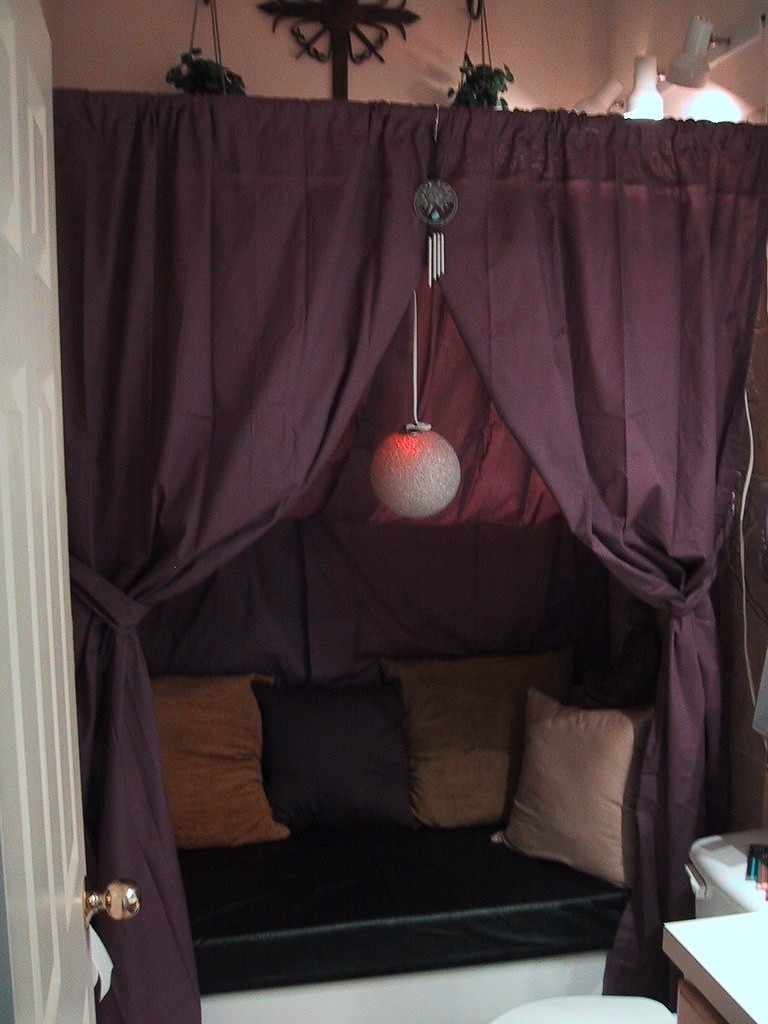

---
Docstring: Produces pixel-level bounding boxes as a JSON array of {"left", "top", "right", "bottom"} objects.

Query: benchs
[{"left": 178, "top": 849, "right": 632, "bottom": 1024}]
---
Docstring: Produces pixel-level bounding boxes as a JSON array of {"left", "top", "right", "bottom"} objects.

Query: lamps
[
  {"left": 371, "top": 290, "right": 461, "bottom": 515},
  {"left": 665, "top": 15, "right": 730, "bottom": 92},
  {"left": 628, "top": 55, "right": 668, "bottom": 122}
]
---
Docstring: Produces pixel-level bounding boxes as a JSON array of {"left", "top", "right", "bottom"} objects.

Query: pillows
[
  {"left": 253, "top": 672, "right": 415, "bottom": 830},
  {"left": 492, "top": 686, "right": 655, "bottom": 889},
  {"left": 150, "top": 673, "right": 290, "bottom": 848},
  {"left": 378, "top": 652, "right": 567, "bottom": 830}
]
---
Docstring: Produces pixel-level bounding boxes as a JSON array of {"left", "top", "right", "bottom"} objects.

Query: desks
[{"left": 662, "top": 912, "right": 768, "bottom": 1024}]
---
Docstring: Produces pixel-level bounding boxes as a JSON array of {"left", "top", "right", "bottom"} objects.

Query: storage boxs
[{"left": 684, "top": 828, "right": 768, "bottom": 920}]
